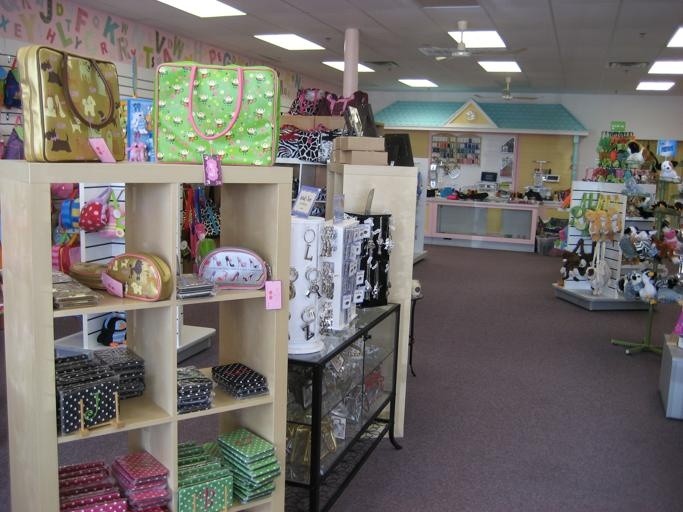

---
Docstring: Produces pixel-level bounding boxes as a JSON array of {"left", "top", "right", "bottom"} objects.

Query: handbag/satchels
[
  {"left": 289, "top": 86, "right": 324, "bottom": 115},
  {"left": 559, "top": 239, "right": 595, "bottom": 281},
  {"left": 198, "top": 246, "right": 272, "bottom": 292},
  {"left": 107, "top": 250, "right": 174, "bottom": 304},
  {"left": 193, "top": 188, "right": 227, "bottom": 240},
  {"left": 153, "top": 60, "right": 282, "bottom": 167},
  {"left": 290, "top": 416, "right": 337, "bottom": 465},
  {"left": 342, "top": 90, "right": 378, "bottom": 139},
  {"left": 18, "top": 42, "right": 130, "bottom": 161},
  {"left": 586, "top": 242, "right": 612, "bottom": 295}
]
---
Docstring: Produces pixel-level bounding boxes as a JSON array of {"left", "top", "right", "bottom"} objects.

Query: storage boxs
[
  {"left": 658, "top": 333, "right": 683, "bottom": 421},
  {"left": 280, "top": 115, "right": 315, "bottom": 131},
  {"left": 315, "top": 116, "right": 348, "bottom": 130}
]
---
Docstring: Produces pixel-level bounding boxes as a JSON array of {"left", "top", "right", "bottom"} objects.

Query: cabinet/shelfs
[
  {"left": 325, "top": 162, "right": 418, "bottom": 439},
  {"left": 285, "top": 302, "right": 402, "bottom": 512},
  {"left": 0, "top": 160, "right": 293, "bottom": 512},
  {"left": 423, "top": 197, "right": 539, "bottom": 252},
  {"left": 552, "top": 180, "right": 659, "bottom": 312}
]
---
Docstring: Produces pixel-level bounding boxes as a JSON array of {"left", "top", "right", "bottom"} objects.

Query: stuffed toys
[
  {"left": 560, "top": 238, "right": 610, "bottom": 295},
  {"left": 626, "top": 273, "right": 643, "bottom": 300},
  {"left": 591, "top": 167, "right": 624, "bottom": 184},
  {"left": 663, "top": 226, "right": 680, "bottom": 260},
  {"left": 620, "top": 227, "right": 638, "bottom": 263},
  {"left": 659, "top": 193, "right": 683, "bottom": 216},
  {"left": 639, "top": 197, "right": 655, "bottom": 219},
  {"left": 634, "top": 230, "right": 661, "bottom": 263},
  {"left": 658, "top": 279, "right": 683, "bottom": 306},
  {"left": 639, "top": 270, "right": 657, "bottom": 306},
  {"left": 661, "top": 162, "right": 680, "bottom": 179},
  {"left": 617, "top": 275, "right": 629, "bottom": 291},
  {"left": 627, "top": 194, "right": 641, "bottom": 218},
  {"left": 570, "top": 194, "right": 621, "bottom": 244},
  {"left": 624, "top": 180, "right": 640, "bottom": 195}
]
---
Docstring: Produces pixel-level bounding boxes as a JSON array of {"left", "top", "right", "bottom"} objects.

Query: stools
[{"left": 408, "top": 280, "right": 424, "bottom": 377}]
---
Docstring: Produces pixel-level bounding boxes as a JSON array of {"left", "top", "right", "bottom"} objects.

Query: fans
[
  {"left": 481, "top": 76, "right": 544, "bottom": 101},
  {"left": 417, "top": 19, "right": 527, "bottom": 64}
]
